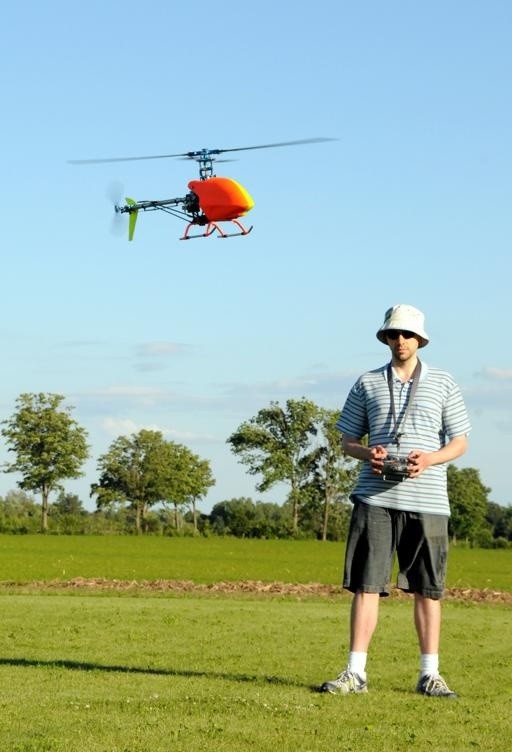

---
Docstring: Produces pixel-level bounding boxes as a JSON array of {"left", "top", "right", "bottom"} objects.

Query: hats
[{"left": 376, "top": 304, "right": 429, "bottom": 348}]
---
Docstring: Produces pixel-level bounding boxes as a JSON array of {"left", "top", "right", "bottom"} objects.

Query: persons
[{"left": 317, "top": 302, "right": 474, "bottom": 697}]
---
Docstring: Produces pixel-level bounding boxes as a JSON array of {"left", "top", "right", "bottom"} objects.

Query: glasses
[{"left": 386, "top": 330, "right": 414, "bottom": 339}]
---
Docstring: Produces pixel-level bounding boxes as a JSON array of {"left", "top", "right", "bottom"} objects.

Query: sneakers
[
  {"left": 320, "top": 670, "right": 367, "bottom": 695},
  {"left": 415, "top": 675, "right": 455, "bottom": 696}
]
